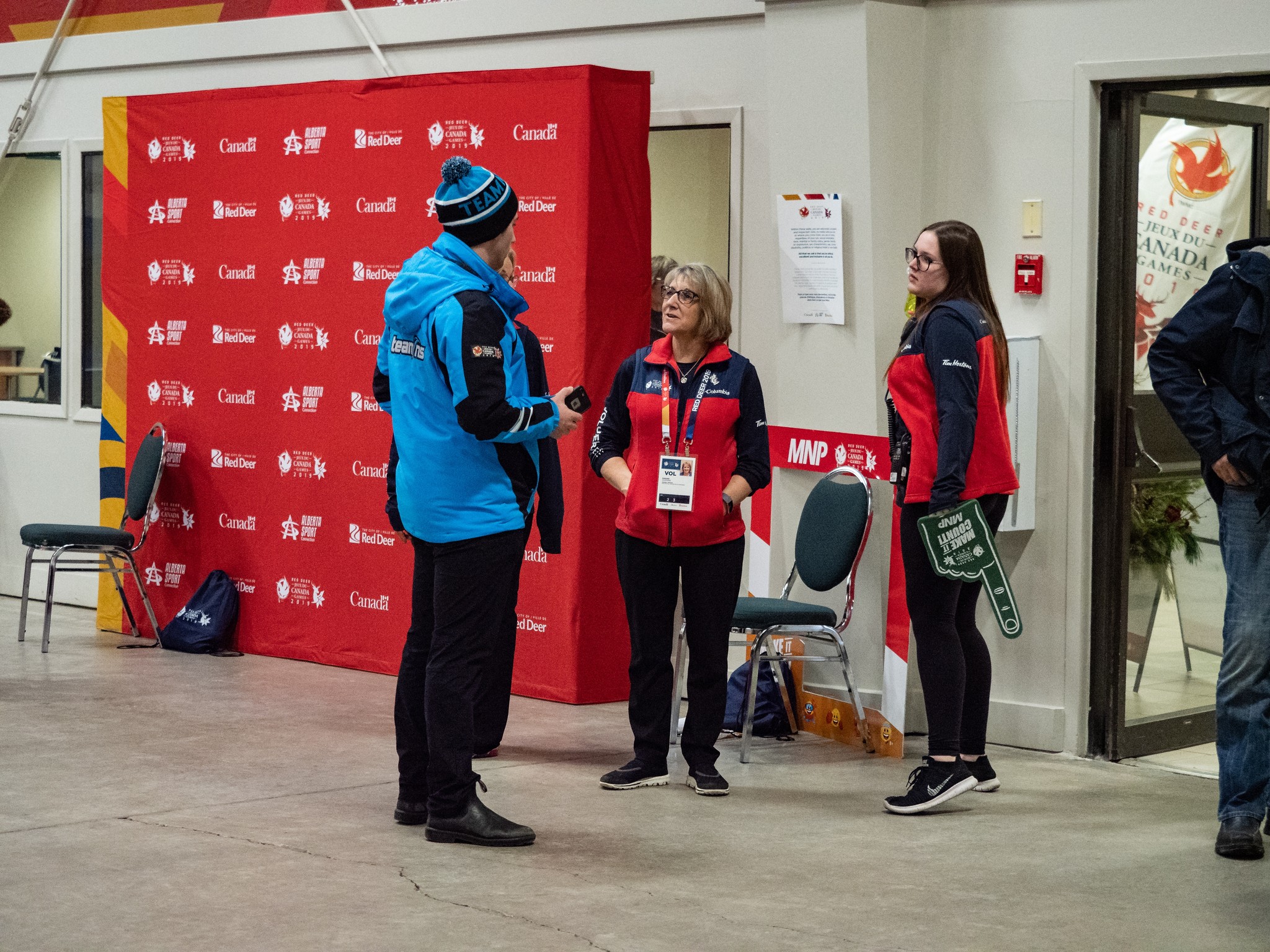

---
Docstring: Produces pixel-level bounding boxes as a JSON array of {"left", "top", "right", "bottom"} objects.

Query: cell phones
[{"left": 564, "top": 385, "right": 592, "bottom": 415}]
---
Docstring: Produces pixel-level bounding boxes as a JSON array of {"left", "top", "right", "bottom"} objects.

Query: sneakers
[
  {"left": 883, "top": 756, "right": 978, "bottom": 814},
  {"left": 959, "top": 754, "right": 1000, "bottom": 793}
]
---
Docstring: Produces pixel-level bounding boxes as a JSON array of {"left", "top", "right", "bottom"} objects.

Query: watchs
[{"left": 721, "top": 493, "right": 733, "bottom": 514}]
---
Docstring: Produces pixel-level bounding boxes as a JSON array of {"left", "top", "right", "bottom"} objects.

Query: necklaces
[{"left": 674, "top": 345, "right": 708, "bottom": 383}]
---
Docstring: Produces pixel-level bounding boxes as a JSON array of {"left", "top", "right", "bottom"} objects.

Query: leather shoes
[
  {"left": 392, "top": 797, "right": 427, "bottom": 825},
  {"left": 1214, "top": 814, "right": 1267, "bottom": 858},
  {"left": 426, "top": 795, "right": 536, "bottom": 847}
]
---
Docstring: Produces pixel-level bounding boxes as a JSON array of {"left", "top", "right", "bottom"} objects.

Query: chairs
[
  {"left": 668, "top": 465, "right": 876, "bottom": 765},
  {"left": 17, "top": 423, "right": 169, "bottom": 655}
]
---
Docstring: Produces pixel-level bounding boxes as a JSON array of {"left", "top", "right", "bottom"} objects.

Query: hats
[{"left": 433, "top": 156, "right": 519, "bottom": 247}]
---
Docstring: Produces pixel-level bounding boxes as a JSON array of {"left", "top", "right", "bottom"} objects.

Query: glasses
[
  {"left": 500, "top": 273, "right": 519, "bottom": 287},
  {"left": 660, "top": 285, "right": 698, "bottom": 305},
  {"left": 905, "top": 246, "right": 947, "bottom": 272}
]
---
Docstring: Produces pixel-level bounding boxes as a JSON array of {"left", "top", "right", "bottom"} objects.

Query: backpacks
[
  {"left": 721, "top": 648, "right": 797, "bottom": 737},
  {"left": 157, "top": 571, "right": 237, "bottom": 655}
]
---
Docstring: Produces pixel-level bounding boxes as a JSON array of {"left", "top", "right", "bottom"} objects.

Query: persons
[
  {"left": 679, "top": 460, "right": 692, "bottom": 477},
  {"left": 371, "top": 154, "right": 583, "bottom": 847},
  {"left": 649, "top": 255, "right": 681, "bottom": 347},
  {"left": 588, "top": 261, "right": 771, "bottom": 797},
  {"left": 1148, "top": 236, "right": 1268, "bottom": 862},
  {"left": 884, "top": 219, "right": 1019, "bottom": 813},
  {"left": 382, "top": 244, "right": 567, "bottom": 762}
]
